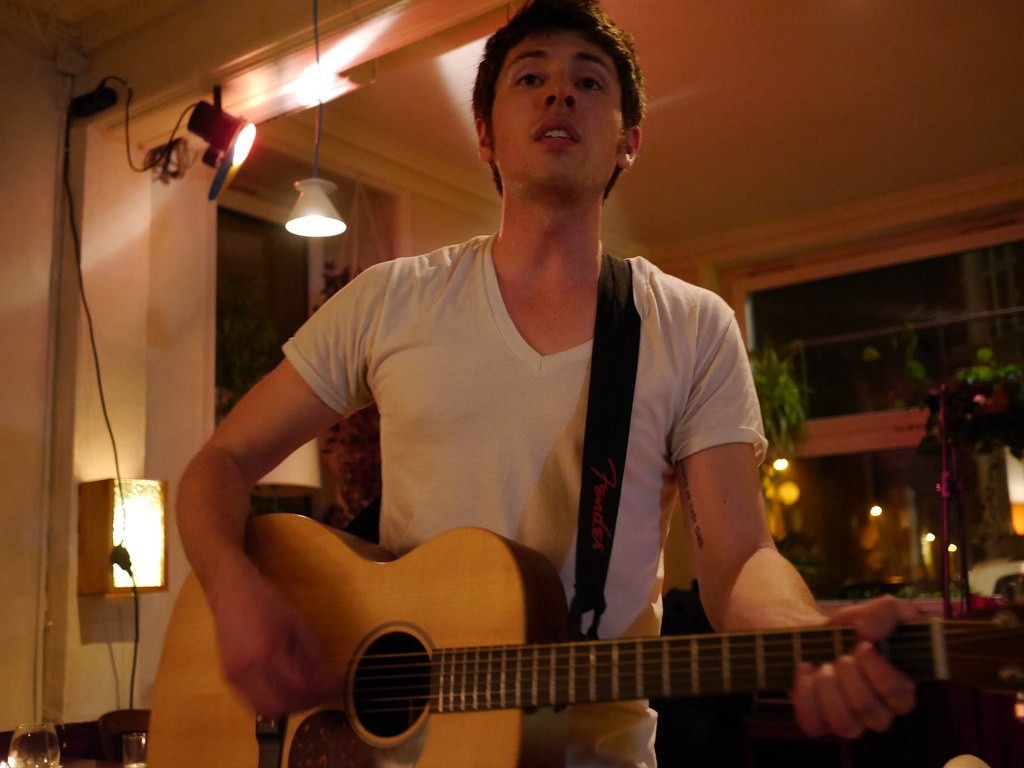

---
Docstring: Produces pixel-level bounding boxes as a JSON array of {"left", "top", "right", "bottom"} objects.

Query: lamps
[
  {"left": 186, "top": 99, "right": 257, "bottom": 168},
  {"left": 285, "top": 0, "right": 347, "bottom": 238}
]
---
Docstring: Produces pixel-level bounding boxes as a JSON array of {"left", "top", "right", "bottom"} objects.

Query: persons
[{"left": 175, "top": 0, "right": 918, "bottom": 768}]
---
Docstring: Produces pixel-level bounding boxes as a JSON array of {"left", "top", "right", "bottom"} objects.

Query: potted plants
[{"left": 909, "top": 346, "right": 1024, "bottom": 460}]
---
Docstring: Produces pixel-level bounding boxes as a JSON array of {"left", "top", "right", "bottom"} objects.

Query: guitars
[{"left": 146, "top": 508, "right": 1024, "bottom": 768}]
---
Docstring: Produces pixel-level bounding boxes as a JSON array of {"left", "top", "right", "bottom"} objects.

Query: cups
[
  {"left": 7, "top": 721, "right": 60, "bottom": 768},
  {"left": 122, "top": 732, "right": 147, "bottom": 768}
]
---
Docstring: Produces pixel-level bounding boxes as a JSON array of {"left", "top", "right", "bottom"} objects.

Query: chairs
[{"left": 743, "top": 694, "right": 854, "bottom": 768}]
